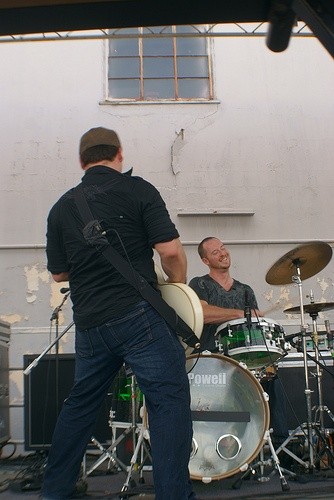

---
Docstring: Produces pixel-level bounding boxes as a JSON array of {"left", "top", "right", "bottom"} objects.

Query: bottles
[{"left": 293, "top": 432, "right": 334, "bottom": 472}]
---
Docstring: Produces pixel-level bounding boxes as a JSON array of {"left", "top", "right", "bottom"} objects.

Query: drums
[
  {"left": 212, "top": 316, "right": 289, "bottom": 370},
  {"left": 148, "top": 351, "right": 270, "bottom": 483}
]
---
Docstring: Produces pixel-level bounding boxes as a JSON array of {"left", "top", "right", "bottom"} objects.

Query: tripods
[
  {"left": 85, "top": 363, "right": 151, "bottom": 500},
  {"left": 232, "top": 258, "right": 334, "bottom": 492}
]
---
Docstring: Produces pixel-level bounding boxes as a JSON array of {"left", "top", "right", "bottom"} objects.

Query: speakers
[{"left": 23, "top": 353, "right": 126, "bottom": 451}]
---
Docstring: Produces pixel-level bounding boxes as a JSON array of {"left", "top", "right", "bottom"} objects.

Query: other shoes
[{"left": 39, "top": 478, "right": 88, "bottom": 500}]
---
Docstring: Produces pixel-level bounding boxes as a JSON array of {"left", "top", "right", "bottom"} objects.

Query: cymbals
[
  {"left": 265, "top": 241, "right": 334, "bottom": 288},
  {"left": 282, "top": 302, "right": 334, "bottom": 315}
]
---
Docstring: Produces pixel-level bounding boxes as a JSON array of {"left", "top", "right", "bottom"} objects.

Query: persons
[
  {"left": 41, "top": 128, "right": 197, "bottom": 500},
  {"left": 189, "top": 237, "right": 308, "bottom": 483}
]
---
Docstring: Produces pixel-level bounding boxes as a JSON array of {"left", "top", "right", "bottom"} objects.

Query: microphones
[
  {"left": 60, "top": 287, "right": 70, "bottom": 294},
  {"left": 285, "top": 331, "right": 302, "bottom": 342},
  {"left": 244, "top": 290, "right": 251, "bottom": 317}
]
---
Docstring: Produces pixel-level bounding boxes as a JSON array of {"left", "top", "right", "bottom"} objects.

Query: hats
[{"left": 80, "top": 127, "right": 120, "bottom": 154}]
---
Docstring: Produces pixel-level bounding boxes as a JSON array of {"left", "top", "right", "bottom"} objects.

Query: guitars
[{"left": 154, "top": 260, "right": 204, "bottom": 358}]
